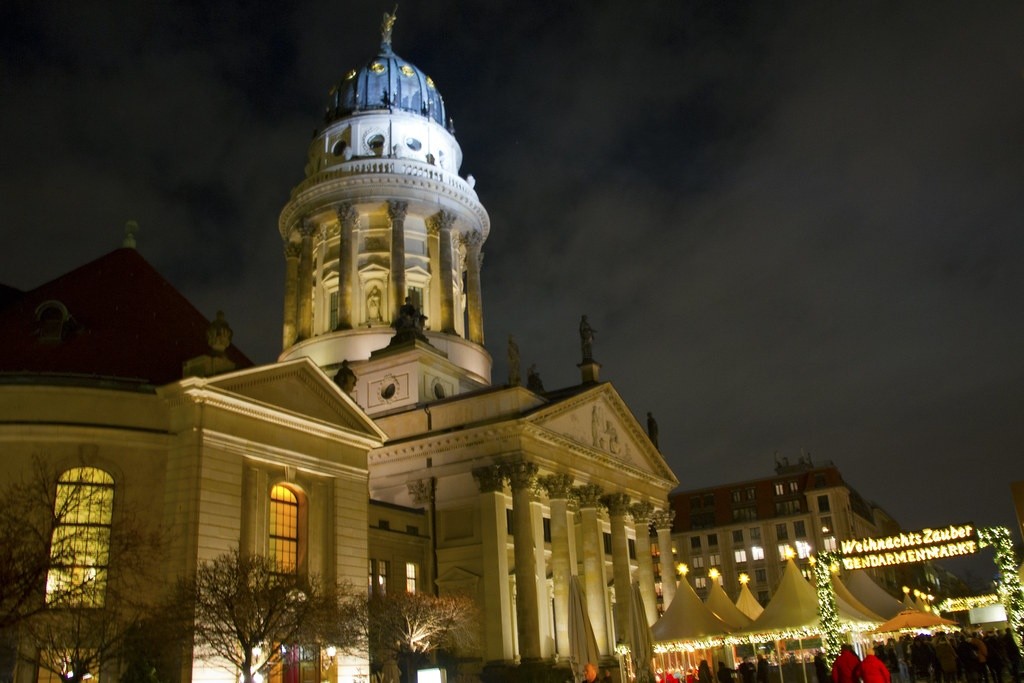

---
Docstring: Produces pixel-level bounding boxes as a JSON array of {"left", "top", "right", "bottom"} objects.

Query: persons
[
  {"left": 698, "top": 628, "right": 1024, "bottom": 683},
  {"left": 334, "top": 360, "right": 358, "bottom": 395},
  {"left": 205, "top": 309, "right": 233, "bottom": 354},
  {"left": 389, "top": 295, "right": 814, "bottom": 478}
]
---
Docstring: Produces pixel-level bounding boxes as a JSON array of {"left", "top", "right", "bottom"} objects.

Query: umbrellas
[{"left": 861, "top": 605, "right": 961, "bottom": 640}]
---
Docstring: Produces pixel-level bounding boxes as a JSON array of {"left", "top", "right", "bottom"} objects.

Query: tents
[{"left": 564, "top": 551, "right": 924, "bottom": 682}]
